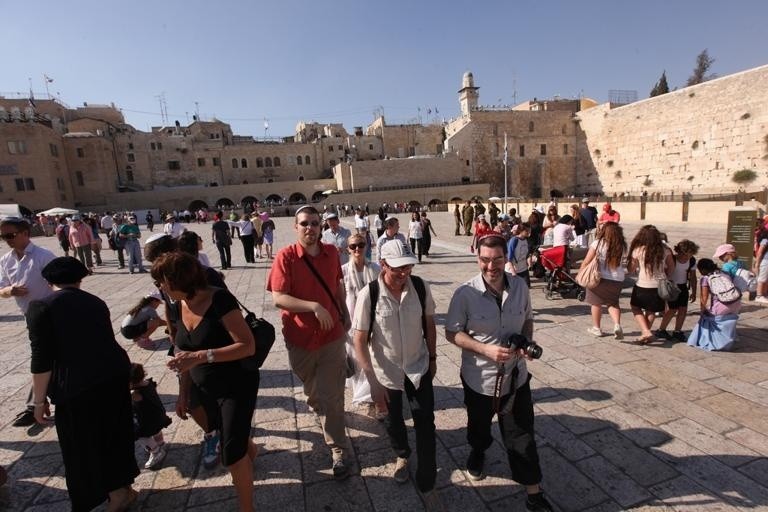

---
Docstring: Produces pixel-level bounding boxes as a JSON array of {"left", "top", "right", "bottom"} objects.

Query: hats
[
  {"left": 534, "top": 206, "right": 543, "bottom": 212},
  {"left": 113, "top": 214, "right": 122, "bottom": 219},
  {"left": 479, "top": 214, "right": 519, "bottom": 233},
  {"left": 166, "top": 214, "right": 175, "bottom": 220},
  {"left": 604, "top": 204, "right": 612, "bottom": 211},
  {"left": 713, "top": 244, "right": 734, "bottom": 259},
  {"left": 381, "top": 239, "right": 417, "bottom": 268},
  {"left": 152, "top": 293, "right": 164, "bottom": 301},
  {"left": 581, "top": 198, "right": 589, "bottom": 203},
  {"left": 71, "top": 215, "right": 81, "bottom": 220}
]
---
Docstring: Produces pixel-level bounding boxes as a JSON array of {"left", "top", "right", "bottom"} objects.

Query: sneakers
[
  {"left": 466, "top": 448, "right": 485, "bottom": 479},
  {"left": 394, "top": 458, "right": 409, "bottom": 482},
  {"left": 130, "top": 270, "right": 135, "bottom": 275},
  {"left": 227, "top": 265, "right": 231, "bottom": 268},
  {"left": 419, "top": 251, "right": 428, "bottom": 264},
  {"left": 141, "top": 270, "right": 148, "bottom": 274},
  {"left": 89, "top": 268, "right": 96, "bottom": 273},
  {"left": 203, "top": 430, "right": 220, "bottom": 463},
  {"left": 755, "top": 295, "right": 767, "bottom": 302},
  {"left": 97, "top": 263, "right": 104, "bottom": 266},
  {"left": 135, "top": 337, "right": 155, "bottom": 348},
  {"left": 455, "top": 232, "right": 471, "bottom": 236},
  {"left": 416, "top": 471, "right": 436, "bottom": 492},
  {"left": 222, "top": 266, "right": 227, "bottom": 269},
  {"left": 588, "top": 324, "right": 687, "bottom": 345},
  {"left": 333, "top": 460, "right": 348, "bottom": 479},
  {"left": 246, "top": 254, "right": 275, "bottom": 263},
  {"left": 525, "top": 496, "right": 553, "bottom": 511},
  {"left": 145, "top": 439, "right": 169, "bottom": 469},
  {"left": 119, "top": 265, "right": 126, "bottom": 270},
  {"left": 12, "top": 406, "right": 36, "bottom": 426}
]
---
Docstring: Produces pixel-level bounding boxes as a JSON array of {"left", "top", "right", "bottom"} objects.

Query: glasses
[
  {"left": 299, "top": 221, "right": 320, "bottom": 226},
  {"left": 479, "top": 257, "right": 504, "bottom": 263},
  {"left": 0, "top": 232, "right": 21, "bottom": 239},
  {"left": 349, "top": 243, "right": 366, "bottom": 249},
  {"left": 384, "top": 264, "right": 413, "bottom": 272}
]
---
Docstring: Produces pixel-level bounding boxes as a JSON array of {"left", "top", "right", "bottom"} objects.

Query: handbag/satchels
[
  {"left": 658, "top": 279, "right": 681, "bottom": 301},
  {"left": 576, "top": 255, "right": 601, "bottom": 288},
  {"left": 109, "top": 239, "right": 116, "bottom": 250},
  {"left": 243, "top": 313, "right": 275, "bottom": 367},
  {"left": 251, "top": 229, "right": 259, "bottom": 242},
  {"left": 533, "top": 256, "right": 547, "bottom": 278}
]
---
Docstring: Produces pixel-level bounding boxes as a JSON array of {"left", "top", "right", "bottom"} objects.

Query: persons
[
  {"left": 126, "top": 362, "right": 173, "bottom": 469},
  {"left": 138, "top": 232, "right": 225, "bottom": 453},
  {"left": 444, "top": 228, "right": 556, "bottom": 512},
  {"left": 150, "top": 252, "right": 262, "bottom": 512},
  {"left": 430, "top": 356, "right": 436, "bottom": 363},
  {"left": 35, "top": 198, "right": 768, "bottom": 351},
  {"left": 0, "top": 216, "right": 56, "bottom": 428},
  {"left": 264, "top": 206, "right": 354, "bottom": 482},
  {"left": 25, "top": 254, "right": 140, "bottom": 510},
  {"left": 355, "top": 238, "right": 439, "bottom": 494}
]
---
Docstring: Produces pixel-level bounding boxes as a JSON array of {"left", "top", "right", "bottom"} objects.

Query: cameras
[{"left": 507, "top": 333, "right": 542, "bottom": 359}]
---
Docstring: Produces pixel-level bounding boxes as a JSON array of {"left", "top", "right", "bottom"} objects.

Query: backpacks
[
  {"left": 707, "top": 271, "right": 741, "bottom": 305},
  {"left": 734, "top": 268, "right": 757, "bottom": 292},
  {"left": 55, "top": 225, "right": 67, "bottom": 241}
]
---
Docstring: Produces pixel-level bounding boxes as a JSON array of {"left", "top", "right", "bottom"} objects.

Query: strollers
[{"left": 536, "top": 245, "right": 586, "bottom": 301}]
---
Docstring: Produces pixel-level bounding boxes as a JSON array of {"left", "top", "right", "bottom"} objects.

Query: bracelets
[
  {"left": 206, "top": 349, "right": 215, "bottom": 365},
  {"left": 31, "top": 401, "right": 48, "bottom": 407}
]
